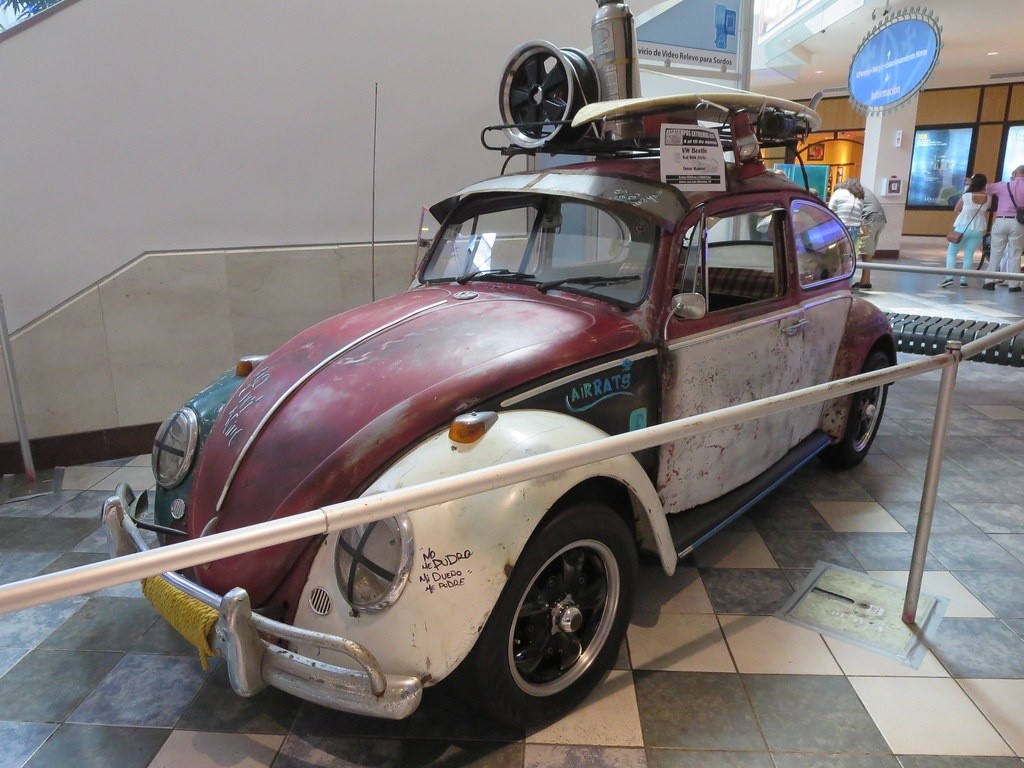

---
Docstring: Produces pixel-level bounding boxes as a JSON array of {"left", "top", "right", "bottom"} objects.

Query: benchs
[{"left": 617, "top": 261, "right": 816, "bottom": 301}]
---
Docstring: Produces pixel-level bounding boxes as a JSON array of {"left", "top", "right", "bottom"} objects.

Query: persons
[
  {"left": 937, "top": 174, "right": 992, "bottom": 287},
  {"left": 834, "top": 180, "right": 887, "bottom": 288},
  {"left": 964, "top": 165, "right": 1024, "bottom": 292},
  {"left": 828, "top": 182, "right": 865, "bottom": 247}
]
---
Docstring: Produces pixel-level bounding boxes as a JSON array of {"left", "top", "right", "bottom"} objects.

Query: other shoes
[
  {"left": 983, "top": 282, "right": 997, "bottom": 290},
  {"left": 938, "top": 280, "right": 955, "bottom": 288},
  {"left": 1009, "top": 286, "right": 1022, "bottom": 292},
  {"left": 961, "top": 282, "right": 968, "bottom": 288},
  {"left": 852, "top": 281, "right": 874, "bottom": 290}
]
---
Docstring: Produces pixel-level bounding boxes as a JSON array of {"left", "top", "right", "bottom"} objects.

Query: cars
[{"left": 97, "top": 0, "right": 898, "bottom": 720}]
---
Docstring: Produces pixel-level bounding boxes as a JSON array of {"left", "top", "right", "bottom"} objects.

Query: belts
[{"left": 996, "top": 216, "right": 1016, "bottom": 218}]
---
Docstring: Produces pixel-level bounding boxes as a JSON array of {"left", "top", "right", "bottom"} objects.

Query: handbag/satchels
[
  {"left": 1017, "top": 206, "right": 1024, "bottom": 223},
  {"left": 946, "top": 230, "right": 963, "bottom": 244}
]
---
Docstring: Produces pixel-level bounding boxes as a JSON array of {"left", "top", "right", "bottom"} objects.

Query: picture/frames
[{"left": 807, "top": 144, "right": 824, "bottom": 161}]
